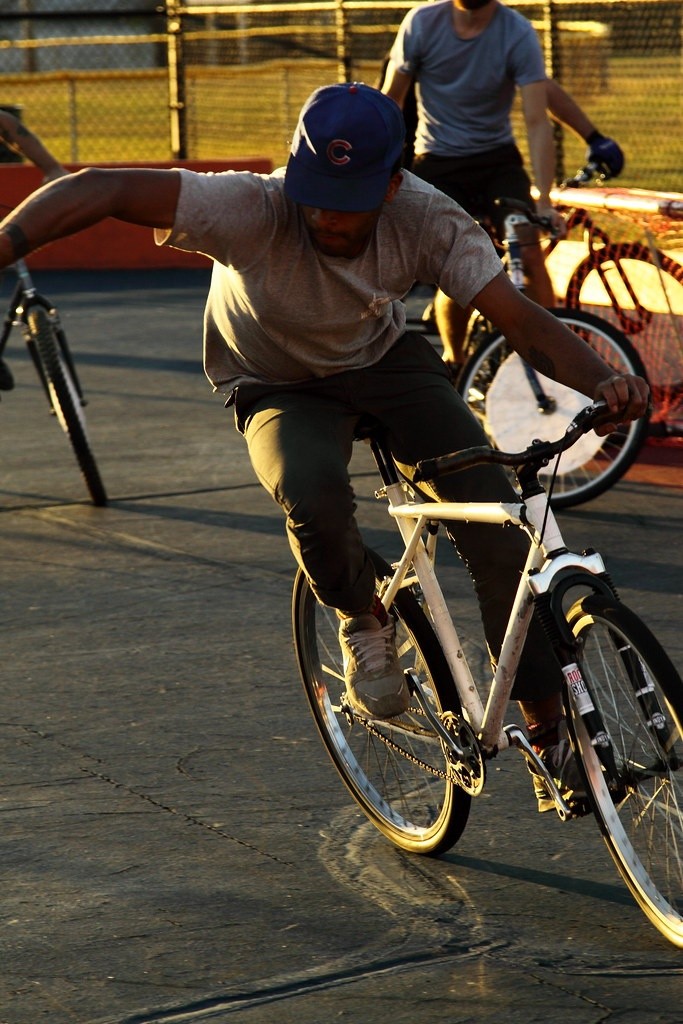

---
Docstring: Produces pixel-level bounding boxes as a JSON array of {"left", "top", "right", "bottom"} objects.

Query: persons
[
  {"left": 0, "top": 109, "right": 74, "bottom": 394},
  {"left": 0, "top": 82, "right": 661, "bottom": 822},
  {"left": 378, "top": 52, "right": 625, "bottom": 185},
  {"left": 380, "top": 1, "right": 569, "bottom": 388}
]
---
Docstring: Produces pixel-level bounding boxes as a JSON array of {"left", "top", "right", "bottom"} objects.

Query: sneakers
[
  {"left": 532, "top": 770, "right": 614, "bottom": 813},
  {"left": 336, "top": 613, "right": 411, "bottom": 722},
  {"left": 0, "top": 359, "right": 15, "bottom": 391}
]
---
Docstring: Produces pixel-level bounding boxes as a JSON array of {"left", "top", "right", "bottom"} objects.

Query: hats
[{"left": 282, "top": 79, "right": 407, "bottom": 213}]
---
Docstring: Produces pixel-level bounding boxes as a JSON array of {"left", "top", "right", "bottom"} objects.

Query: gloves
[{"left": 585, "top": 130, "right": 625, "bottom": 182}]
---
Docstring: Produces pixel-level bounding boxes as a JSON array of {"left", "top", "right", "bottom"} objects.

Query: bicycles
[{"left": 1, "top": 158, "right": 683, "bottom": 951}]
